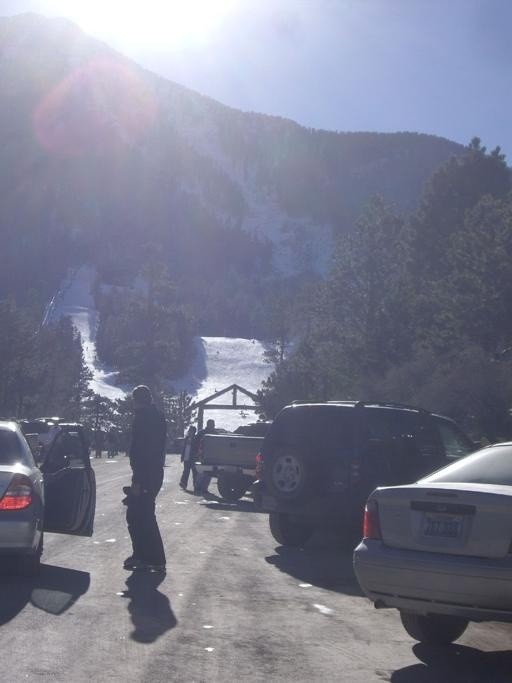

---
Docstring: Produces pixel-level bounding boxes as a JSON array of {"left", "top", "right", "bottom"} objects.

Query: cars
[
  {"left": 0, "top": 420, "right": 98, "bottom": 579},
  {"left": 350, "top": 442, "right": 512, "bottom": 645},
  {"left": 17, "top": 416, "right": 92, "bottom": 461}
]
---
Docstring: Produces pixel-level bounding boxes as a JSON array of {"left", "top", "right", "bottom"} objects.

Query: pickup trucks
[{"left": 194, "top": 420, "right": 272, "bottom": 509}]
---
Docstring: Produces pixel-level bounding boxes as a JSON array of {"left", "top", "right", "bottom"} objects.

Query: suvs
[{"left": 251, "top": 398, "right": 479, "bottom": 561}]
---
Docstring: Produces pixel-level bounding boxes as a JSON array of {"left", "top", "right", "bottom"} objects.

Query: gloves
[{"left": 122, "top": 486, "right": 155, "bottom": 505}]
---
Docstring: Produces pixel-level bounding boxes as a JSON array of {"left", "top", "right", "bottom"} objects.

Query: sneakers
[{"left": 125, "top": 555, "right": 164, "bottom": 568}]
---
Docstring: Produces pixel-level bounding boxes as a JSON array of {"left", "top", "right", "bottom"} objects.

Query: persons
[
  {"left": 47, "top": 419, "right": 117, "bottom": 459},
  {"left": 192, "top": 418, "right": 226, "bottom": 494},
  {"left": 178, "top": 424, "right": 199, "bottom": 489},
  {"left": 119, "top": 384, "right": 166, "bottom": 572}
]
